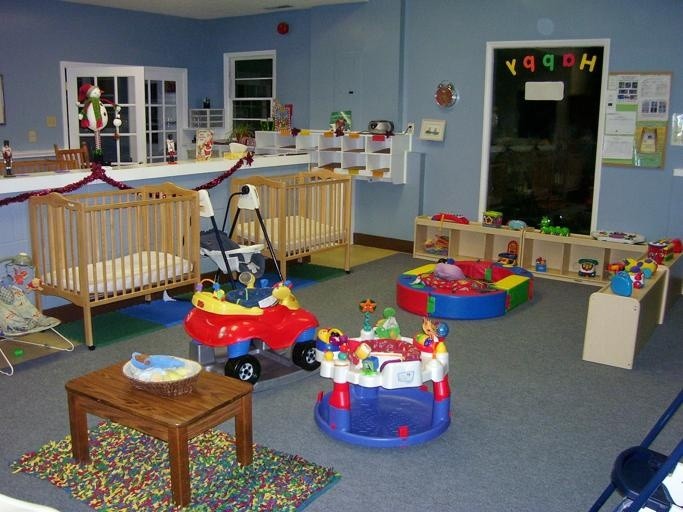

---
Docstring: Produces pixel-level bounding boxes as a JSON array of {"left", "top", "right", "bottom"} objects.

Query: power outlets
[{"left": 407, "top": 122, "right": 414, "bottom": 136}]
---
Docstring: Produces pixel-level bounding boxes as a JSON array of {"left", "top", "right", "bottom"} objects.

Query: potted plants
[{"left": 226, "top": 123, "right": 255, "bottom": 146}]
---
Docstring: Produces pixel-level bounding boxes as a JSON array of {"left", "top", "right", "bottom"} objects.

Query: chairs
[
  {"left": 53, "top": 140, "right": 91, "bottom": 170},
  {"left": 588, "top": 389, "right": 683, "bottom": 512}
]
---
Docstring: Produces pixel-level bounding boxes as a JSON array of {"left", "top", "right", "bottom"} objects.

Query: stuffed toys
[{"left": 76, "top": 84, "right": 108, "bottom": 132}]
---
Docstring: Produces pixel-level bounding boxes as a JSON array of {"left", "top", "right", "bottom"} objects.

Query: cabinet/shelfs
[
  {"left": 521, "top": 228, "right": 648, "bottom": 287},
  {"left": 254, "top": 129, "right": 411, "bottom": 185},
  {"left": 412, "top": 215, "right": 535, "bottom": 268},
  {"left": 582, "top": 250, "right": 683, "bottom": 370}
]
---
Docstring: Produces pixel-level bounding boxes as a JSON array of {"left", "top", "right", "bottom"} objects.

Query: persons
[
  {"left": 2, "top": 140, "right": 16, "bottom": 176},
  {"left": 166, "top": 133, "right": 177, "bottom": 164}
]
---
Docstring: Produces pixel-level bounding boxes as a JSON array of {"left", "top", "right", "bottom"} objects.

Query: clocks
[{"left": 434, "top": 79, "right": 459, "bottom": 110}]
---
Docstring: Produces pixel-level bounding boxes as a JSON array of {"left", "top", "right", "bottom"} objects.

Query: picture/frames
[
  {"left": 0, "top": 73, "right": 6, "bottom": 126},
  {"left": 478, "top": 38, "right": 611, "bottom": 237},
  {"left": 419, "top": 119, "right": 446, "bottom": 142}
]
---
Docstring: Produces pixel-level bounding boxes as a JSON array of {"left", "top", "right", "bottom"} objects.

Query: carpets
[{"left": 8, "top": 419, "right": 342, "bottom": 512}]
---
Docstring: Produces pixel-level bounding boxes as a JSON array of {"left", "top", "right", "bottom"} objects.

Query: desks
[{"left": 64, "top": 354, "right": 254, "bottom": 507}]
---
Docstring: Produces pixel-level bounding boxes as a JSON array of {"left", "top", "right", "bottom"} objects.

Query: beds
[
  {"left": 230, "top": 168, "right": 352, "bottom": 281},
  {"left": 28, "top": 180, "right": 201, "bottom": 350}
]
